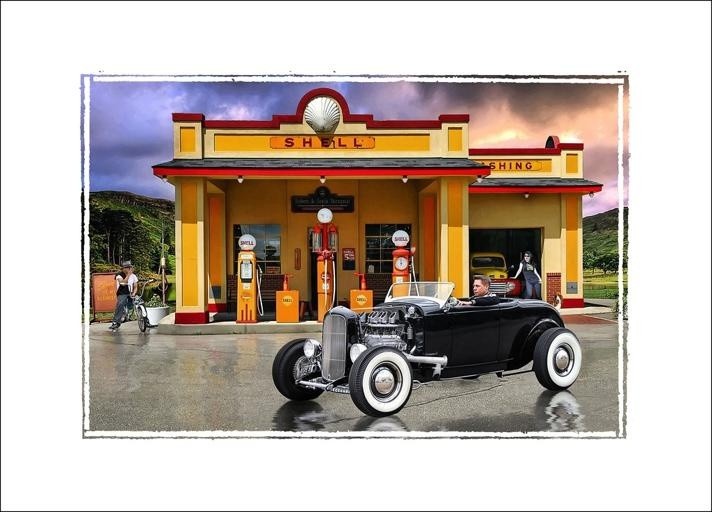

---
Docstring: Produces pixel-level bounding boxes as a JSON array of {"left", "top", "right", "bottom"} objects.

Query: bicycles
[{"left": 120, "top": 278, "right": 154, "bottom": 333}]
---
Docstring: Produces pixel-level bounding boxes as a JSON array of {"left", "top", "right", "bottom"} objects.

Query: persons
[
  {"left": 456, "top": 275, "right": 502, "bottom": 305},
  {"left": 510, "top": 251, "right": 543, "bottom": 299},
  {"left": 108, "top": 261, "right": 155, "bottom": 329}
]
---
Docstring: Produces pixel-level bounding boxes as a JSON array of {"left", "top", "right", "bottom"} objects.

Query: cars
[
  {"left": 368, "top": 238, "right": 380, "bottom": 248},
  {"left": 271, "top": 278, "right": 584, "bottom": 419},
  {"left": 275, "top": 390, "right": 584, "bottom": 432},
  {"left": 468, "top": 253, "right": 523, "bottom": 300}
]
